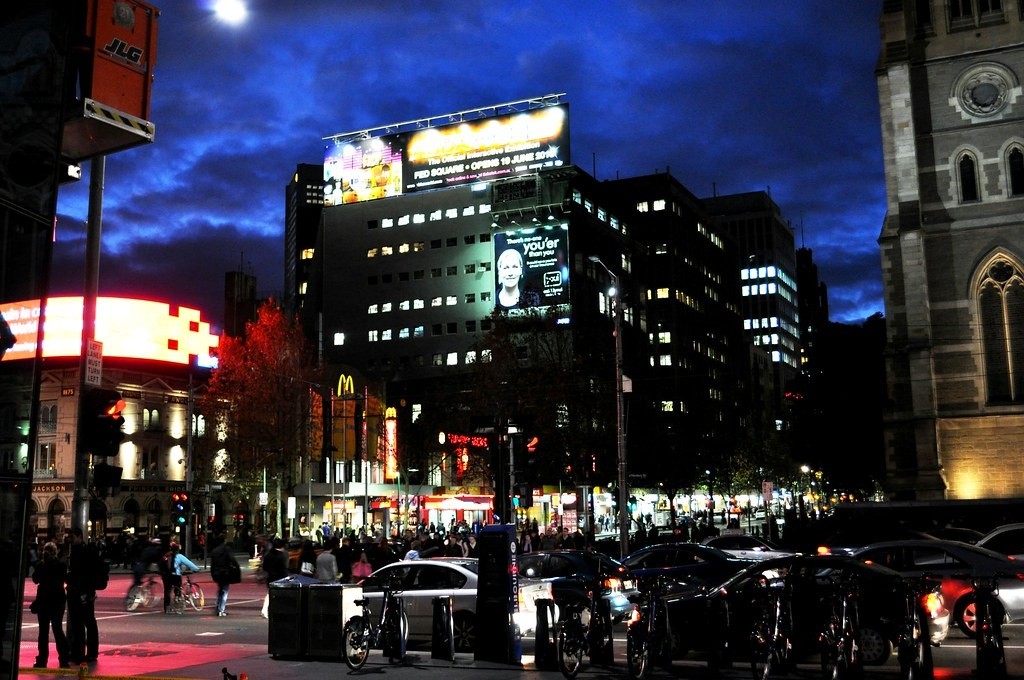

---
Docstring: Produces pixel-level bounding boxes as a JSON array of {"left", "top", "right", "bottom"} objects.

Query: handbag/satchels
[
  {"left": 352, "top": 554, "right": 371, "bottom": 577},
  {"left": 82, "top": 546, "right": 110, "bottom": 589},
  {"left": 301, "top": 561, "right": 315, "bottom": 575},
  {"left": 225, "top": 562, "right": 241, "bottom": 584}
]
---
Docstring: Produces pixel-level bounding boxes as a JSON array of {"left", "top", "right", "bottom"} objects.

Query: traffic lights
[
  {"left": 610, "top": 487, "right": 619, "bottom": 511},
  {"left": 90, "top": 386, "right": 125, "bottom": 457},
  {"left": 172, "top": 492, "right": 190, "bottom": 525},
  {"left": 232, "top": 513, "right": 247, "bottom": 528}
]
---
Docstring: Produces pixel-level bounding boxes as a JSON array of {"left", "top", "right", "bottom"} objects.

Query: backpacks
[{"left": 158, "top": 552, "right": 178, "bottom": 576}]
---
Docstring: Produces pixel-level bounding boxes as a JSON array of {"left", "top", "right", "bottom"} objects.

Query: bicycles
[
  {"left": 557, "top": 571, "right": 613, "bottom": 680},
  {"left": 951, "top": 569, "right": 1019, "bottom": 680},
  {"left": 810, "top": 571, "right": 871, "bottom": 680},
  {"left": 626, "top": 575, "right": 670, "bottom": 680},
  {"left": 747, "top": 567, "right": 808, "bottom": 680},
  {"left": 888, "top": 570, "right": 946, "bottom": 680},
  {"left": 169, "top": 569, "right": 205, "bottom": 616},
  {"left": 124, "top": 570, "right": 163, "bottom": 612},
  {"left": 342, "top": 573, "right": 411, "bottom": 670}
]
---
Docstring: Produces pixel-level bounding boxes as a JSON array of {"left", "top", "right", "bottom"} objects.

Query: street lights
[{"left": 588, "top": 255, "right": 628, "bottom": 560}]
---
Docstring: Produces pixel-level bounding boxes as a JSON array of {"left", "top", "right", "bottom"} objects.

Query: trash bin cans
[
  {"left": 302, "top": 584, "right": 364, "bottom": 657},
  {"left": 267, "top": 574, "right": 307, "bottom": 656}
]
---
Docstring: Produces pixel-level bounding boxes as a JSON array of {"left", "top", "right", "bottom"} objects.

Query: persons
[
  {"left": 261, "top": 538, "right": 289, "bottom": 618},
  {"left": 519, "top": 517, "right": 583, "bottom": 553},
  {"left": 495, "top": 249, "right": 539, "bottom": 310},
  {"left": 113, "top": 531, "right": 199, "bottom": 612},
  {"left": 210, "top": 533, "right": 234, "bottom": 616},
  {"left": 598, "top": 514, "right": 610, "bottom": 531},
  {"left": 298, "top": 519, "right": 477, "bottom": 584},
  {"left": 30, "top": 528, "right": 99, "bottom": 668},
  {"left": 635, "top": 512, "right": 659, "bottom": 552},
  {"left": 679, "top": 509, "right": 708, "bottom": 543}
]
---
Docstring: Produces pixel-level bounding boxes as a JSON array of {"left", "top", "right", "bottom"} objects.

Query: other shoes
[
  {"left": 33, "top": 662, "right": 47, "bottom": 668},
  {"left": 218, "top": 612, "right": 227, "bottom": 616},
  {"left": 59, "top": 662, "right": 71, "bottom": 669}
]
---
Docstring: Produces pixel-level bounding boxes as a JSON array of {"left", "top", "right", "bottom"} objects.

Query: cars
[
  {"left": 352, "top": 557, "right": 559, "bottom": 652},
  {"left": 644, "top": 550, "right": 953, "bottom": 661},
  {"left": 713, "top": 512, "right": 722, "bottom": 524},
  {"left": 756, "top": 509, "right": 766, "bottom": 520},
  {"left": 518, "top": 520, "right": 1024, "bottom": 626}
]
